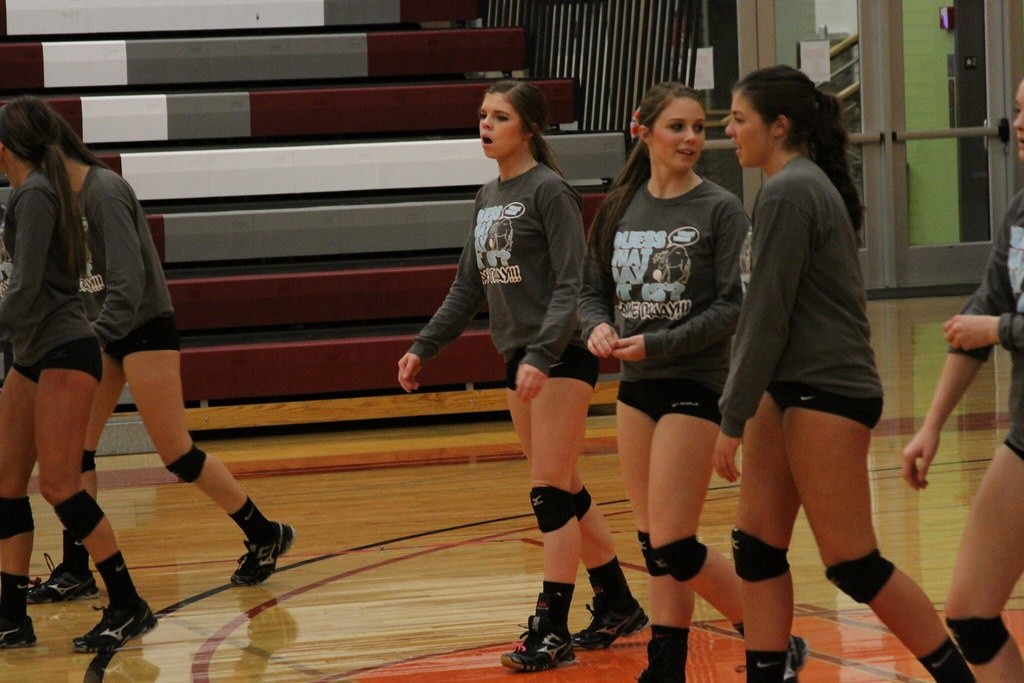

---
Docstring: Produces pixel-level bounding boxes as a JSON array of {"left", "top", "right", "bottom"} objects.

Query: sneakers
[
  {"left": 72, "top": 600, "right": 157, "bottom": 653},
  {"left": 573, "top": 597, "right": 650, "bottom": 650},
  {"left": 231, "top": 521, "right": 295, "bottom": 587},
  {"left": 27, "top": 563, "right": 100, "bottom": 604},
  {"left": 784, "top": 634, "right": 809, "bottom": 683},
  {"left": 0, "top": 616, "right": 36, "bottom": 648},
  {"left": 502, "top": 615, "right": 576, "bottom": 672}
]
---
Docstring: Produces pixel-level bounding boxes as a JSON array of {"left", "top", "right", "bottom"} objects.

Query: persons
[
  {"left": 0, "top": 95, "right": 297, "bottom": 654},
  {"left": 903, "top": 78, "right": 1024, "bottom": 683},
  {"left": 715, "top": 64, "right": 978, "bottom": 683},
  {"left": 577, "top": 83, "right": 808, "bottom": 683},
  {"left": 398, "top": 80, "right": 649, "bottom": 670}
]
